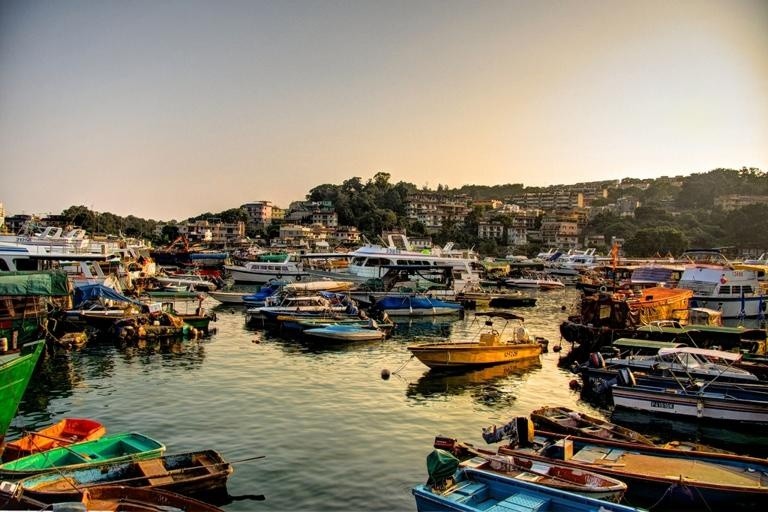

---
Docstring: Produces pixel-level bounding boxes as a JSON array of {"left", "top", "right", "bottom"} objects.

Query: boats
[
  {"left": 406, "top": 312, "right": 543, "bottom": 367},
  {"left": 571, "top": 346, "right": 768, "bottom": 424}
]
